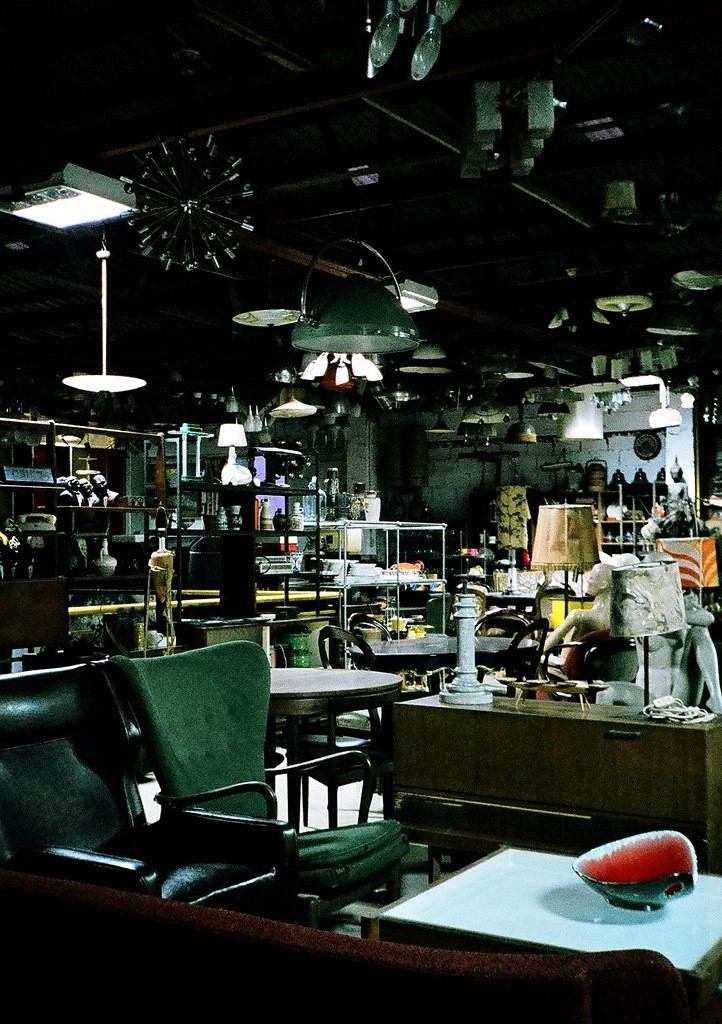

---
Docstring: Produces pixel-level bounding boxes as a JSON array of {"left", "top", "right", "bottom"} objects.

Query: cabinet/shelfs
[
  {"left": 305, "top": 519, "right": 448, "bottom": 671},
  {"left": 568, "top": 482, "right": 657, "bottom": 555},
  {"left": 142, "top": 434, "right": 323, "bottom": 617},
  {"left": 382, "top": 694, "right": 722, "bottom": 884}
]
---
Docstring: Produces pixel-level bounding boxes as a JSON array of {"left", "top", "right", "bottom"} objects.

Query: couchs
[{"left": 0, "top": 869, "right": 691, "bottom": 1024}]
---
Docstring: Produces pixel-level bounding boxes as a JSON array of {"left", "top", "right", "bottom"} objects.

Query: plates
[{"left": 348, "top": 563, "right": 423, "bottom": 584}]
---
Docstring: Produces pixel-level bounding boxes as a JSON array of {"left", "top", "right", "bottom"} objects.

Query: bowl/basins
[{"left": 571, "top": 829, "right": 697, "bottom": 912}]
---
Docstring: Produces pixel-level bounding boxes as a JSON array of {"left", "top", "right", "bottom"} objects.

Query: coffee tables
[{"left": 360, "top": 845, "right": 722, "bottom": 1024}]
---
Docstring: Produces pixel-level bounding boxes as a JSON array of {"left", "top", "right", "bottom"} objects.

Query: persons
[
  {"left": 668, "top": 455, "right": 692, "bottom": 508},
  {"left": 543, "top": 563, "right": 722, "bottom": 715},
  {"left": 59, "top": 475, "right": 119, "bottom": 508}
]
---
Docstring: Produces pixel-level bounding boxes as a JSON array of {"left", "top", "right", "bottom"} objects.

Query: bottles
[
  {"left": 363, "top": 490, "right": 381, "bottom": 522},
  {"left": 303, "top": 481, "right": 328, "bottom": 522},
  {"left": 273, "top": 508, "right": 287, "bottom": 531},
  {"left": 216, "top": 506, "right": 228, "bottom": 530},
  {"left": 607, "top": 532, "right": 614, "bottom": 542},
  {"left": 624, "top": 531, "right": 633, "bottom": 542},
  {"left": 91, "top": 538, "right": 117, "bottom": 577},
  {"left": 229, "top": 505, "right": 242, "bottom": 531},
  {"left": 290, "top": 502, "right": 305, "bottom": 532},
  {"left": 258, "top": 498, "right": 273, "bottom": 530}
]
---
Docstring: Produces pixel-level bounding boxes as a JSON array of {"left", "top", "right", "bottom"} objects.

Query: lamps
[
  {"left": 528, "top": 503, "right": 600, "bottom": 620},
  {"left": 608, "top": 560, "right": 687, "bottom": 723},
  {"left": 656, "top": 536, "right": 719, "bottom": 608},
  {"left": 269, "top": 390, "right": 318, "bottom": 419},
  {"left": 536, "top": 401, "right": 571, "bottom": 422},
  {"left": 61, "top": 228, "right": 148, "bottom": 394},
  {"left": 424, "top": 410, "right": 455, "bottom": 436},
  {"left": 505, "top": 402, "right": 539, "bottom": 445},
  {"left": 495, "top": 484, "right": 529, "bottom": 596},
  {"left": 218, "top": 423, "right": 247, "bottom": 465},
  {"left": 648, "top": 401, "right": 682, "bottom": 430},
  {"left": 290, "top": 236, "right": 422, "bottom": 356},
  {"left": 461, "top": 398, "right": 510, "bottom": 425},
  {"left": 369, "top": 0, "right": 462, "bottom": 81}
]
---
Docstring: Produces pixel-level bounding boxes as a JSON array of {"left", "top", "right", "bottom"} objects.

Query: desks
[
  {"left": 269, "top": 666, "right": 404, "bottom": 824},
  {"left": 175, "top": 616, "right": 335, "bottom": 666},
  {"left": 350, "top": 635, "right": 540, "bottom": 700}
]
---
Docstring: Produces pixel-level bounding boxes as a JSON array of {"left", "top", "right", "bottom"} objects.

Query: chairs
[
  {"left": 542, "top": 627, "right": 608, "bottom": 677},
  {"left": 473, "top": 608, "right": 539, "bottom": 640},
  {"left": 109, "top": 639, "right": 409, "bottom": 931},
  {"left": 348, "top": 613, "right": 393, "bottom": 641},
  {"left": 285, "top": 624, "right": 385, "bottom": 829},
  {"left": 0, "top": 660, "right": 290, "bottom": 920},
  {"left": 505, "top": 618, "right": 550, "bottom": 699}
]
